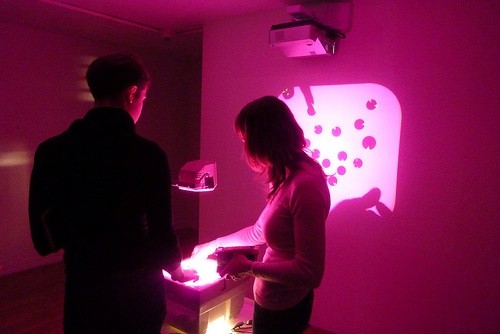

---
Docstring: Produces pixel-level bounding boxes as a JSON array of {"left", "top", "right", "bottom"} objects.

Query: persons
[
  {"left": 28, "top": 51, "right": 199, "bottom": 334},
  {"left": 219, "top": 95, "right": 331, "bottom": 334}
]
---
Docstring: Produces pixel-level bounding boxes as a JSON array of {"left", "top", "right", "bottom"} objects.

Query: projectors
[{"left": 270, "top": 24, "right": 334, "bottom": 56}]
